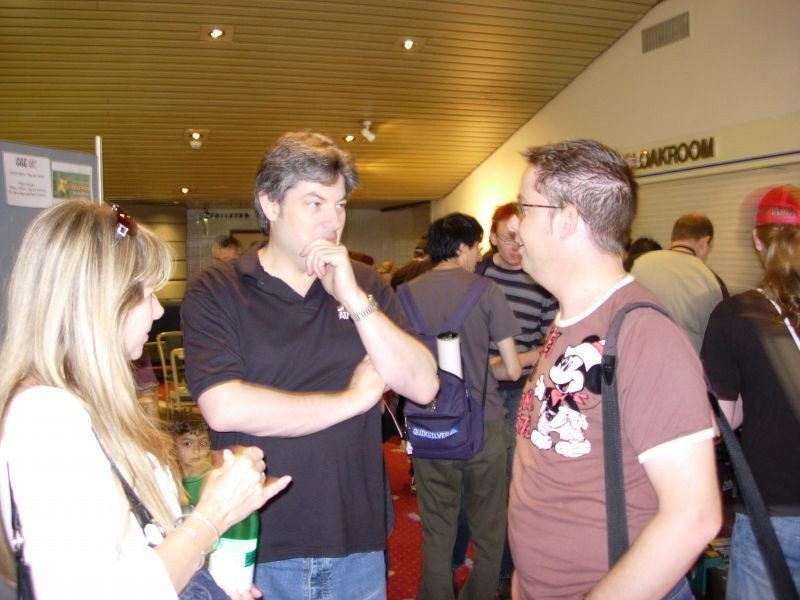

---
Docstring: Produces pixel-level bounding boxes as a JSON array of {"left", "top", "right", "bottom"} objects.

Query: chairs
[{"left": 156, "top": 331, "right": 198, "bottom": 432}]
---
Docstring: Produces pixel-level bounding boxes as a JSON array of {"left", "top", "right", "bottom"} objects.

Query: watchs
[{"left": 351, "top": 294, "right": 378, "bottom": 321}]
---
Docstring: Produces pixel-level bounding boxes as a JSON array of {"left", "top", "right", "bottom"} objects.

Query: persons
[
  {"left": 508, "top": 139, "right": 724, "bottom": 600},
  {"left": 179, "top": 131, "right": 440, "bottom": 600},
  {"left": 0, "top": 129, "right": 800, "bottom": 600}
]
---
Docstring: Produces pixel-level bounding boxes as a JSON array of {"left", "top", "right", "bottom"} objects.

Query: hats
[{"left": 756, "top": 184, "right": 800, "bottom": 225}]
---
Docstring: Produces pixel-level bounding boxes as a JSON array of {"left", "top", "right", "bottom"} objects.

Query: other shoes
[
  {"left": 498, "top": 579, "right": 512, "bottom": 600},
  {"left": 411, "top": 477, "right": 417, "bottom": 493}
]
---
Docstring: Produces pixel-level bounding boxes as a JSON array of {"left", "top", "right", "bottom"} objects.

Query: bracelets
[
  {"left": 172, "top": 513, "right": 219, "bottom": 553},
  {"left": 165, "top": 522, "right": 205, "bottom": 571}
]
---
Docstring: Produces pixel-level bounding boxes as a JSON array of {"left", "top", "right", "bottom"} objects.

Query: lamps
[{"left": 361, "top": 121, "right": 377, "bottom": 143}]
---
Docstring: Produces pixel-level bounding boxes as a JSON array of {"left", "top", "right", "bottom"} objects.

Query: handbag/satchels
[{"left": 6, "top": 428, "right": 229, "bottom": 600}]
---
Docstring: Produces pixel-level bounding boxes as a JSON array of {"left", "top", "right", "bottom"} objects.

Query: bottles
[
  {"left": 436, "top": 331, "right": 462, "bottom": 379},
  {"left": 208, "top": 445, "right": 257, "bottom": 598}
]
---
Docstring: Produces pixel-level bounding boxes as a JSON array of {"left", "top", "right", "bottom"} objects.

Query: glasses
[
  {"left": 516, "top": 203, "right": 591, "bottom": 224},
  {"left": 497, "top": 235, "right": 517, "bottom": 245},
  {"left": 112, "top": 203, "right": 138, "bottom": 246}
]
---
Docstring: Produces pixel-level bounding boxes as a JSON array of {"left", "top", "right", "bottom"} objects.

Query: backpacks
[{"left": 399, "top": 278, "right": 483, "bottom": 460}]
